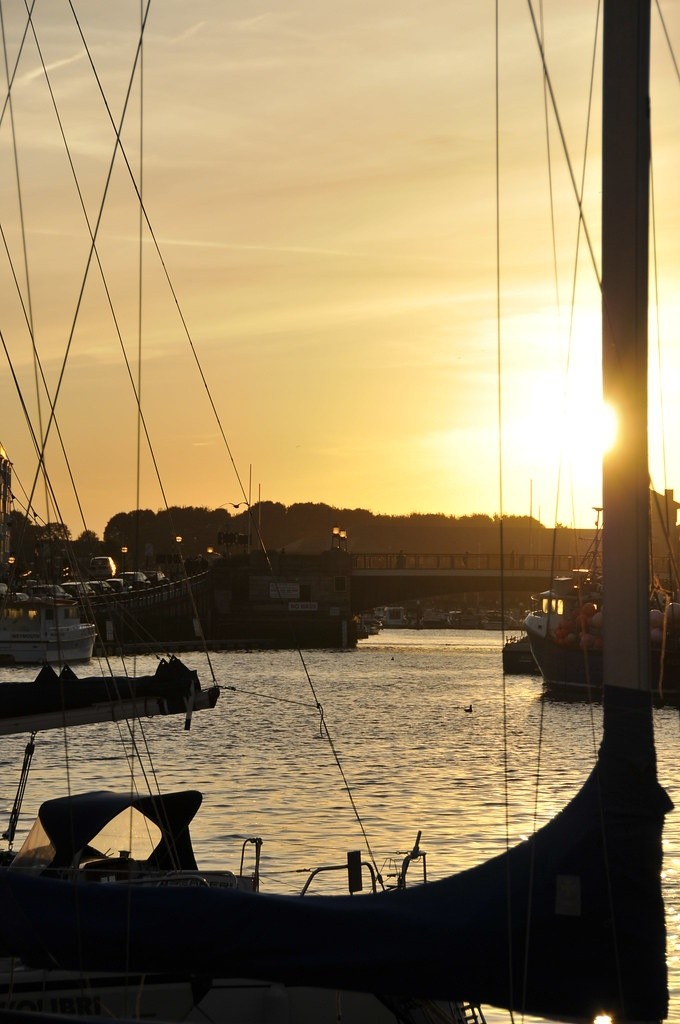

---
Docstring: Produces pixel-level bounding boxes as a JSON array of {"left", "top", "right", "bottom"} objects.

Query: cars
[{"left": 0, "top": 555, "right": 173, "bottom": 602}]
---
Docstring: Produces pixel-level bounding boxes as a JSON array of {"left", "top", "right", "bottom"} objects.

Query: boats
[
  {"left": 0, "top": 791, "right": 484, "bottom": 1024},
  {"left": 355, "top": 484, "right": 680, "bottom": 702},
  {"left": 0, "top": 592, "right": 101, "bottom": 666}
]
